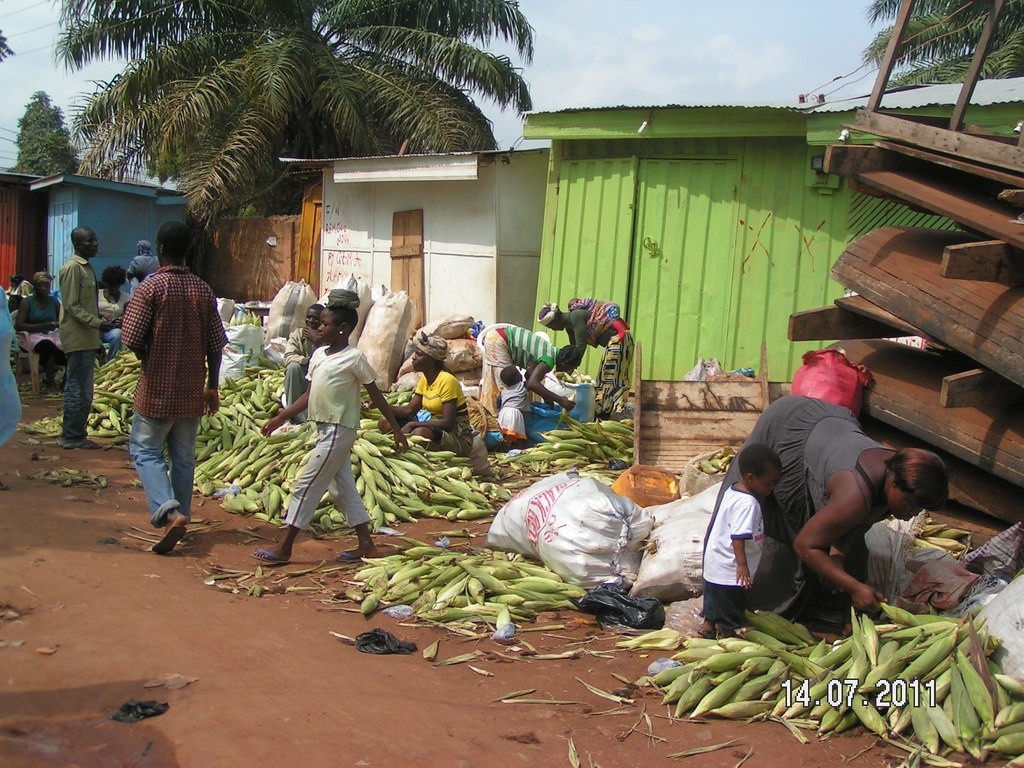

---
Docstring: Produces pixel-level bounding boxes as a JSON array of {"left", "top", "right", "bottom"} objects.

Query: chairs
[{"left": 10, "top": 309, "right": 68, "bottom": 397}]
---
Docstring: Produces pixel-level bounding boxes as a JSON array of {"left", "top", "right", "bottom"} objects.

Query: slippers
[
  {"left": 151, "top": 524, "right": 187, "bottom": 554},
  {"left": 334, "top": 551, "right": 362, "bottom": 562},
  {"left": 250, "top": 549, "right": 289, "bottom": 563},
  {"left": 64, "top": 440, "right": 102, "bottom": 450}
]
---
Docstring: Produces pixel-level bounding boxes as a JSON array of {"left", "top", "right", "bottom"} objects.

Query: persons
[
  {"left": 97, "top": 266, "right": 132, "bottom": 362},
  {"left": 697, "top": 444, "right": 782, "bottom": 637},
  {"left": 6, "top": 274, "right": 33, "bottom": 297},
  {"left": 120, "top": 221, "right": 229, "bottom": 554},
  {"left": 59, "top": 226, "right": 113, "bottom": 450},
  {"left": 702, "top": 394, "right": 949, "bottom": 636},
  {"left": 496, "top": 365, "right": 542, "bottom": 449},
  {"left": 284, "top": 303, "right": 326, "bottom": 424},
  {"left": 127, "top": 240, "right": 161, "bottom": 295},
  {"left": 248, "top": 289, "right": 408, "bottom": 562},
  {"left": 481, "top": 326, "right": 581, "bottom": 416},
  {"left": 537, "top": 298, "right": 634, "bottom": 415},
  {"left": 361, "top": 334, "right": 474, "bottom": 459},
  {"left": 15, "top": 272, "right": 69, "bottom": 393}
]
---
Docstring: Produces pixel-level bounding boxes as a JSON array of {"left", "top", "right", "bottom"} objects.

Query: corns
[
  {"left": 87, "top": 314, "right": 513, "bottom": 537},
  {"left": 694, "top": 446, "right": 738, "bottom": 473},
  {"left": 343, "top": 543, "right": 589, "bottom": 628},
  {"left": 506, "top": 367, "right": 634, "bottom": 471},
  {"left": 907, "top": 518, "right": 974, "bottom": 557},
  {"left": 646, "top": 602, "right": 1024, "bottom": 768}
]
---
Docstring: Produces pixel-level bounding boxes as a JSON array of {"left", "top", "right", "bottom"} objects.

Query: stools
[{"left": 103, "top": 342, "right": 127, "bottom": 363}]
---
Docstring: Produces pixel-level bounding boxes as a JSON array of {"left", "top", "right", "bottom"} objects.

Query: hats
[{"left": 417, "top": 331, "right": 448, "bottom": 360}]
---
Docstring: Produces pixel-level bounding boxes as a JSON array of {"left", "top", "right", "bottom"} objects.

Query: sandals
[
  {"left": 718, "top": 632, "right": 743, "bottom": 639},
  {"left": 696, "top": 628, "right": 715, "bottom": 640}
]
